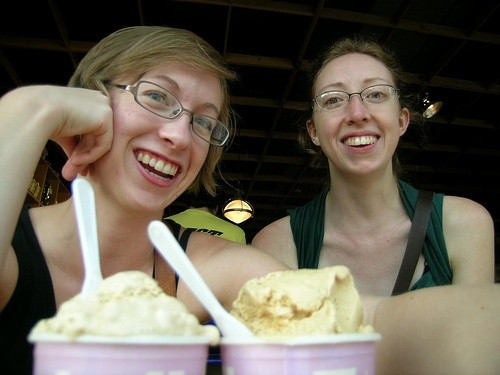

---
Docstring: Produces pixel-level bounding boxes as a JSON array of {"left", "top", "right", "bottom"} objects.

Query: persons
[
  {"left": 251, "top": 38, "right": 495, "bottom": 303},
  {"left": 1, "top": 21, "right": 500, "bottom": 375},
  {"left": 163, "top": 187, "right": 247, "bottom": 246}
]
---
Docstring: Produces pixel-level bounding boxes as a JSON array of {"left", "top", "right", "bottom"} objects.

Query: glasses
[
  {"left": 104, "top": 80, "right": 230, "bottom": 147},
  {"left": 311, "top": 84, "right": 401, "bottom": 112}
]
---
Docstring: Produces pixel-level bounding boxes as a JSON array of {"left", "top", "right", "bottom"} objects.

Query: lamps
[
  {"left": 221, "top": 181, "right": 255, "bottom": 224},
  {"left": 405, "top": 86, "right": 443, "bottom": 119}
]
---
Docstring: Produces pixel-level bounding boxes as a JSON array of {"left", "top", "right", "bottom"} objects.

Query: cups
[
  {"left": 27, "top": 331, "right": 211, "bottom": 375},
  {"left": 219, "top": 333, "right": 381, "bottom": 375}
]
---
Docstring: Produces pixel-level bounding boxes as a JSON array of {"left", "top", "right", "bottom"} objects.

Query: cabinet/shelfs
[{"left": 26, "top": 155, "right": 72, "bottom": 208}]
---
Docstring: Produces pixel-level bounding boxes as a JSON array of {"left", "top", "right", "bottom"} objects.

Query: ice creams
[
  {"left": 219, "top": 263, "right": 379, "bottom": 374},
  {"left": 26, "top": 270, "right": 221, "bottom": 375}
]
